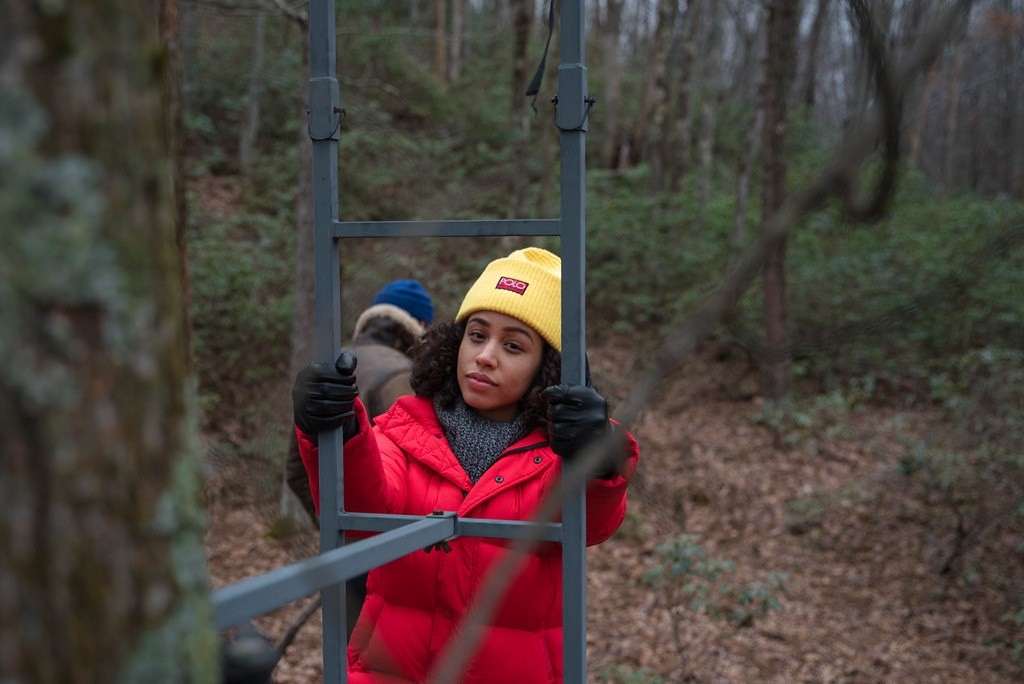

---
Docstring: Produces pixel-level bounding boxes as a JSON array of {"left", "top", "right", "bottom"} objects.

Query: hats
[
  {"left": 454, "top": 247, "right": 562, "bottom": 355},
  {"left": 373, "top": 279, "right": 435, "bottom": 325}
]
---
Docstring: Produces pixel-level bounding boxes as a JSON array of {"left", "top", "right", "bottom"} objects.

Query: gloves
[
  {"left": 540, "top": 384, "right": 618, "bottom": 480},
  {"left": 291, "top": 351, "right": 360, "bottom": 448}
]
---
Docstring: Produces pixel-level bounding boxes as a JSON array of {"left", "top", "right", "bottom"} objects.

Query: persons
[
  {"left": 286, "top": 276, "right": 438, "bottom": 644},
  {"left": 287, "top": 244, "right": 643, "bottom": 684}
]
